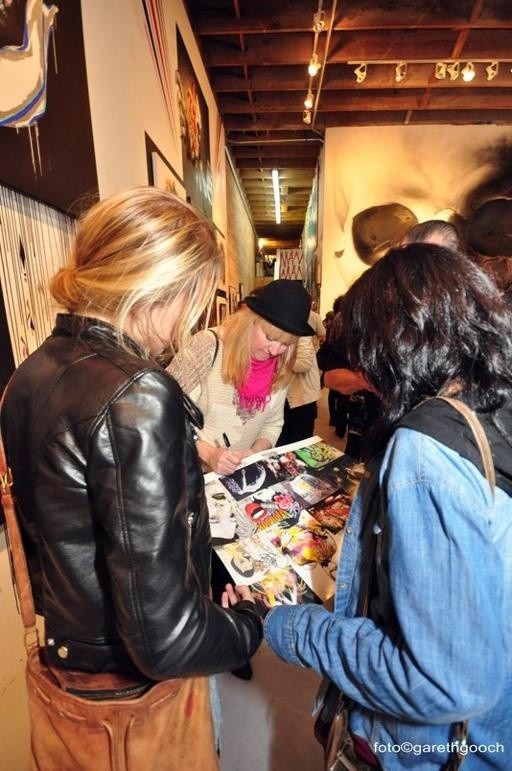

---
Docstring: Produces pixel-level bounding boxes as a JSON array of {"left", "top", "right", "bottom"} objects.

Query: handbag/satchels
[
  {"left": 323, "top": 708, "right": 385, "bottom": 771},
  {"left": 27, "top": 638, "right": 221, "bottom": 771}
]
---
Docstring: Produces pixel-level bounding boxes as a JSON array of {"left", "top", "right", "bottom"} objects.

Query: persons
[
  {"left": 160, "top": 277, "right": 319, "bottom": 679},
  {"left": 250, "top": 283, "right": 322, "bottom": 450},
  {"left": 322, "top": 220, "right": 462, "bottom": 461},
  {"left": 1, "top": 186, "right": 263, "bottom": 768},
  {"left": 316, "top": 294, "right": 348, "bottom": 438},
  {"left": 263, "top": 240, "right": 512, "bottom": 770}
]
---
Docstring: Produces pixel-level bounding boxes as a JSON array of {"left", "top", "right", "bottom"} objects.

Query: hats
[{"left": 244, "top": 279, "right": 315, "bottom": 337}]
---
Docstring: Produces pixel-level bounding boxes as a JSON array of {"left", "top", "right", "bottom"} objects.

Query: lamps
[
  {"left": 302, "top": 11, "right": 324, "bottom": 125},
  {"left": 347, "top": 61, "right": 499, "bottom": 83}
]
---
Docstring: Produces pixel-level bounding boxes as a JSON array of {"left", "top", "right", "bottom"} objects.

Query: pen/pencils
[{"left": 223, "top": 433, "right": 231, "bottom": 450}]
